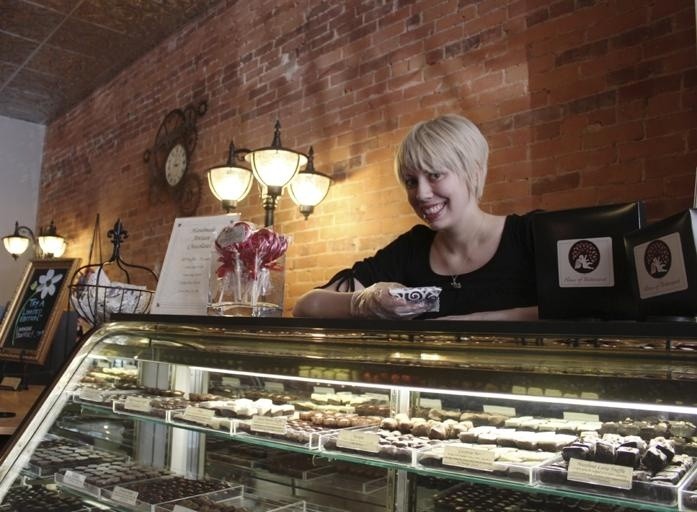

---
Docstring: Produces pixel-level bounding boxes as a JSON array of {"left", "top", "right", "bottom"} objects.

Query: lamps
[
  {"left": 0, "top": 219, "right": 68, "bottom": 261},
  {"left": 200, "top": 116, "right": 334, "bottom": 230}
]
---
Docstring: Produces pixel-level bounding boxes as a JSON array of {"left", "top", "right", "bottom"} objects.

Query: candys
[{"left": 214, "top": 222, "right": 288, "bottom": 308}]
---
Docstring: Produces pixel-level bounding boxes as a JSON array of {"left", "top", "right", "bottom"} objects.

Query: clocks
[{"left": 141, "top": 98, "right": 208, "bottom": 214}]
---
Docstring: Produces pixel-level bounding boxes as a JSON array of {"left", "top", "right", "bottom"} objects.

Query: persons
[{"left": 293, "top": 114, "right": 539, "bottom": 321}]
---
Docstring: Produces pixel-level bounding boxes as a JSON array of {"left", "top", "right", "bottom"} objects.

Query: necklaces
[{"left": 438, "top": 213, "right": 492, "bottom": 289}]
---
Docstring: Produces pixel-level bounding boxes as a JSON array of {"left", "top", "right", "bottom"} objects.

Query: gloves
[{"left": 350, "top": 281, "right": 436, "bottom": 321}]
